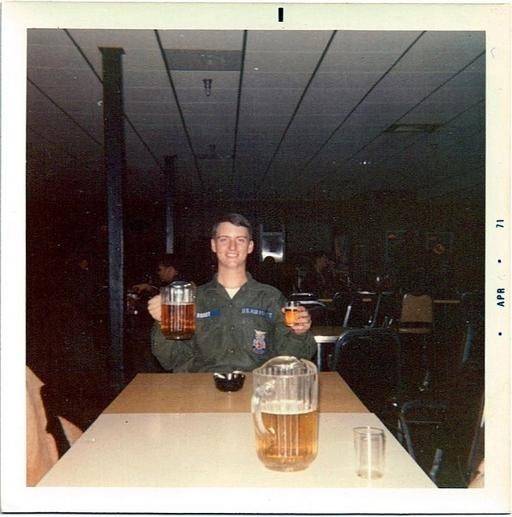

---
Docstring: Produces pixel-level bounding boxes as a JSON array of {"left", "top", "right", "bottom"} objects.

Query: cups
[
  {"left": 284, "top": 300, "right": 299, "bottom": 326},
  {"left": 352, "top": 426, "right": 388, "bottom": 480},
  {"left": 159, "top": 285, "right": 197, "bottom": 339},
  {"left": 249, "top": 367, "right": 320, "bottom": 473}
]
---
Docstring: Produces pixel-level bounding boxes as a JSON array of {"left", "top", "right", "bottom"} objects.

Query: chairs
[
  {"left": 398, "top": 293, "right": 435, "bottom": 369},
  {"left": 342, "top": 289, "right": 383, "bottom": 329},
  {"left": 330, "top": 327, "right": 416, "bottom": 458}
]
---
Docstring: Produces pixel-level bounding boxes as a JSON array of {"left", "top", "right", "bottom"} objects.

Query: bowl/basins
[{"left": 214, "top": 372, "right": 245, "bottom": 392}]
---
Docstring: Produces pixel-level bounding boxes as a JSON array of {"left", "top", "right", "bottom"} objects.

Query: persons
[
  {"left": 126, "top": 253, "right": 198, "bottom": 381},
  {"left": 147, "top": 212, "right": 318, "bottom": 374},
  {"left": 26, "top": 365, "right": 84, "bottom": 490},
  {"left": 299, "top": 250, "right": 342, "bottom": 325},
  {"left": 258, "top": 256, "right": 289, "bottom": 291}
]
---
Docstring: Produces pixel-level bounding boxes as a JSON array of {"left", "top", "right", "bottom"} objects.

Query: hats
[{"left": 155, "top": 255, "right": 180, "bottom": 267}]
